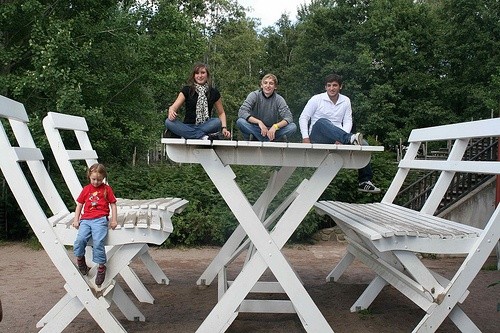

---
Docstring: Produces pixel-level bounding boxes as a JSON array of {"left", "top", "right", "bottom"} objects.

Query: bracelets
[
  {"left": 273, "top": 124, "right": 280, "bottom": 130},
  {"left": 222, "top": 127, "right": 226, "bottom": 129}
]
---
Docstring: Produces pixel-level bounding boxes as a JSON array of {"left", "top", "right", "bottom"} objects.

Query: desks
[{"left": 161, "top": 137, "right": 384, "bottom": 333}]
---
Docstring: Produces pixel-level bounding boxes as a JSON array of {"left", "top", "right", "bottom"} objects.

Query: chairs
[
  {"left": 42, "top": 111, "right": 189, "bottom": 303},
  {"left": 0, "top": 95, "right": 173, "bottom": 333}
]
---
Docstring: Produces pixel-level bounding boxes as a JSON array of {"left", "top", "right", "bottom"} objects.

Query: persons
[
  {"left": 71, "top": 163, "right": 118, "bottom": 286},
  {"left": 298, "top": 74, "right": 381, "bottom": 193},
  {"left": 236, "top": 73, "right": 297, "bottom": 142},
  {"left": 164, "top": 64, "right": 230, "bottom": 140}
]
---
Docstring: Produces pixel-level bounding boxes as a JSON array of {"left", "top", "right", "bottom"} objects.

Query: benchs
[{"left": 314, "top": 118, "right": 500, "bottom": 333}]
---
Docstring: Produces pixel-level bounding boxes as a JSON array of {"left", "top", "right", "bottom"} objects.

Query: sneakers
[
  {"left": 351, "top": 132, "right": 361, "bottom": 145},
  {"left": 358, "top": 181, "right": 381, "bottom": 192}
]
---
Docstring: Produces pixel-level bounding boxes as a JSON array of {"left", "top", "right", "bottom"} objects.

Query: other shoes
[
  {"left": 249, "top": 134, "right": 253, "bottom": 140},
  {"left": 282, "top": 135, "right": 287, "bottom": 142},
  {"left": 78, "top": 256, "right": 88, "bottom": 275},
  {"left": 95, "top": 265, "right": 106, "bottom": 285}
]
